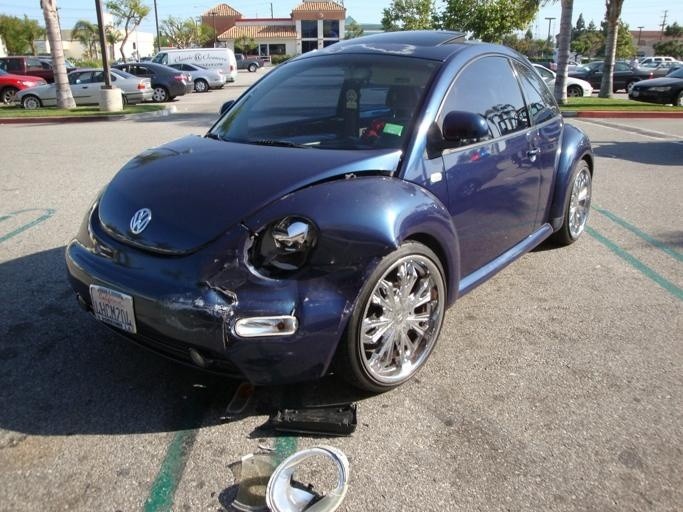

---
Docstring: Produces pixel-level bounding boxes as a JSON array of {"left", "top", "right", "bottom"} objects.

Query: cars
[
  {"left": 429, "top": 100, "right": 563, "bottom": 205},
  {"left": 59, "top": 30, "right": 595, "bottom": 392},
  {"left": 527, "top": 56, "right": 682, "bottom": 109},
  {"left": 0, "top": 47, "right": 265, "bottom": 111}
]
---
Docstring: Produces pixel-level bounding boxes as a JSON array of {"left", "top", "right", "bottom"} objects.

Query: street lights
[
  {"left": 636, "top": 26, "right": 645, "bottom": 46},
  {"left": 194, "top": 20, "right": 200, "bottom": 28},
  {"left": 544, "top": 18, "right": 555, "bottom": 54},
  {"left": 211, "top": 12, "right": 217, "bottom": 47}
]
